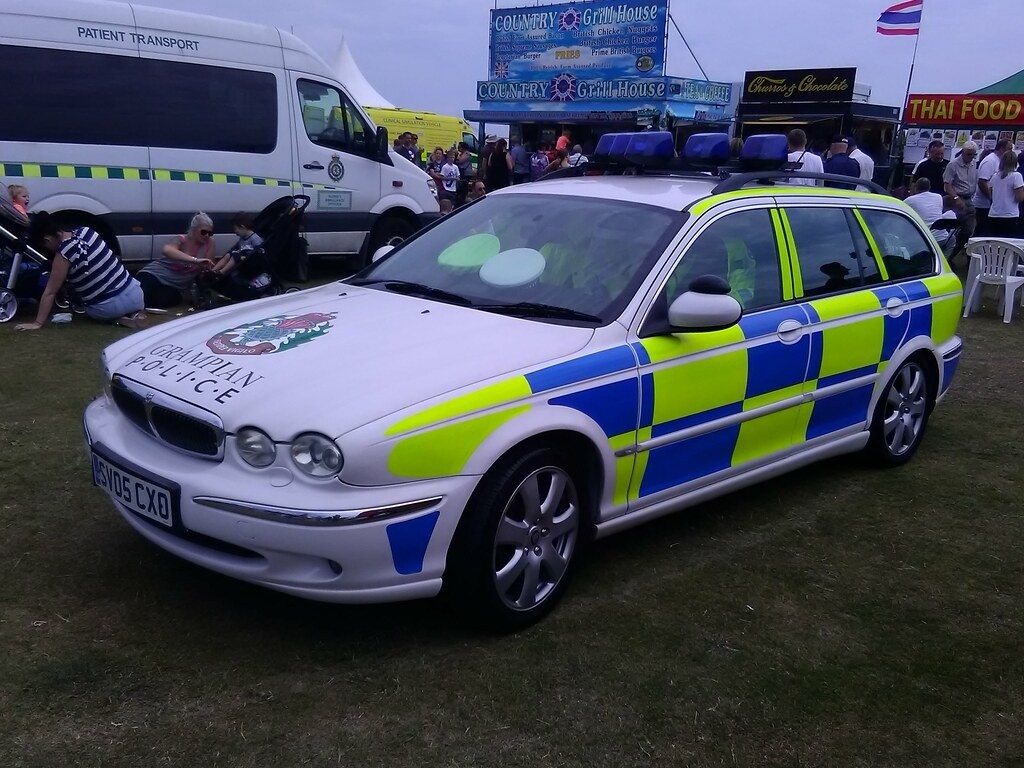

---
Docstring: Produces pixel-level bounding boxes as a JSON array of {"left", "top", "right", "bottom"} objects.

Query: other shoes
[
  {"left": 55, "top": 296, "right": 69, "bottom": 308},
  {"left": 71, "top": 299, "right": 85, "bottom": 314}
]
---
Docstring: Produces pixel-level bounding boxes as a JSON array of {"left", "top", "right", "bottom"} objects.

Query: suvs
[{"left": 78, "top": 130, "right": 962, "bottom": 636}]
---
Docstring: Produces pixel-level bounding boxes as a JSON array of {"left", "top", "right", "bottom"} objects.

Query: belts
[{"left": 957, "top": 194, "right": 971, "bottom": 200}]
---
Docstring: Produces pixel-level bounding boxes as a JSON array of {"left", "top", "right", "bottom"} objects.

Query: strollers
[
  {"left": 0, "top": 187, "right": 87, "bottom": 322},
  {"left": 183, "top": 193, "right": 311, "bottom": 311}
]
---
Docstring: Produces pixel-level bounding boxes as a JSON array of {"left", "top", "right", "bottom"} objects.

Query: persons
[
  {"left": 211, "top": 211, "right": 263, "bottom": 275},
  {"left": 731, "top": 128, "right": 1024, "bottom": 275},
  {"left": 135, "top": 211, "right": 216, "bottom": 308},
  {"left": 8, "top": 184, "right": 29, "bottom": 218},
  {"left": 393, "top": 129, "right": 588, "bottom": 215},
  {"left": 13, "top": 210, "right": 147, "bottom": 332}
]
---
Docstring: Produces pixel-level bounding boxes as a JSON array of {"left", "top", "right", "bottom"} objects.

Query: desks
[{"left": 962, "top": 237, "right": 1024, "bottom": 307}]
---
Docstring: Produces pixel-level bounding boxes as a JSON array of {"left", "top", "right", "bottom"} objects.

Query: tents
[
  {"left": 903, "top": 69, "right": 1024, "bottom": 127},
  {"left": 737, "top": 101, "right": 900, "bottom": 166}
]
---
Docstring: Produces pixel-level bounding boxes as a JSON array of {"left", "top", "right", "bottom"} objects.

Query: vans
[{"left": 0, "top": 2, "right": 440, "bottom": 293}]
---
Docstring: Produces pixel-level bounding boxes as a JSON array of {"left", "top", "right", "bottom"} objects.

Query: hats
[
  {"left": 486, "top": 135, "right": 497, "bottom": 143},
  {"left": 832, "top": 134, "right": 849, "bottom": 143}
]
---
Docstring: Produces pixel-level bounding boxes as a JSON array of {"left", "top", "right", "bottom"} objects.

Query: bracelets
[{"left": 192, "top": 257, "right": 197, "bottom": 264}]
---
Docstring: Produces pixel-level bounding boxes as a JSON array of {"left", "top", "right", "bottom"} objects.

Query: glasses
[
  {"left": 964, "top": 152, "right": 978, "bottom": 156},
  {"left": 413, "top": 138, "right": 418, "bottom": 139},
  {"left": 194, "top": 227, "right": 214, "bottom": 237}
]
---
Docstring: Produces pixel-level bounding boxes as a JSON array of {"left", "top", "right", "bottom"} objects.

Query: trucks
[{"left": 327, "top": 104, "right": 479, "bottom": 164}]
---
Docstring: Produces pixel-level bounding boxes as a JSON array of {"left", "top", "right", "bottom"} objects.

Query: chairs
[
  {"left": 929, "top": 214, "right": 977, "bottom": 263},
  {"left": 963, "top": 240, "right": 1024, "bottom": 323},
  {"left": 664, "top": 228, "right": 756, "bottom": 310},
  {"left": 526, "top": 207, "right": 614, "bottom": 290},
  {"left": 881, "top": 233, "right": 910, "bottom": 260}
]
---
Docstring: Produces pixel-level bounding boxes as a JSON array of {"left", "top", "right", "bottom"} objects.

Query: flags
[{"left": 876, "top": 0, "right": 923, "bottom": 35}]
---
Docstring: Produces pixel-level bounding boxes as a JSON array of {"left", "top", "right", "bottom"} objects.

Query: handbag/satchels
[{"left": 459, "top": 168, "right": 466, "bottom": 177}]
